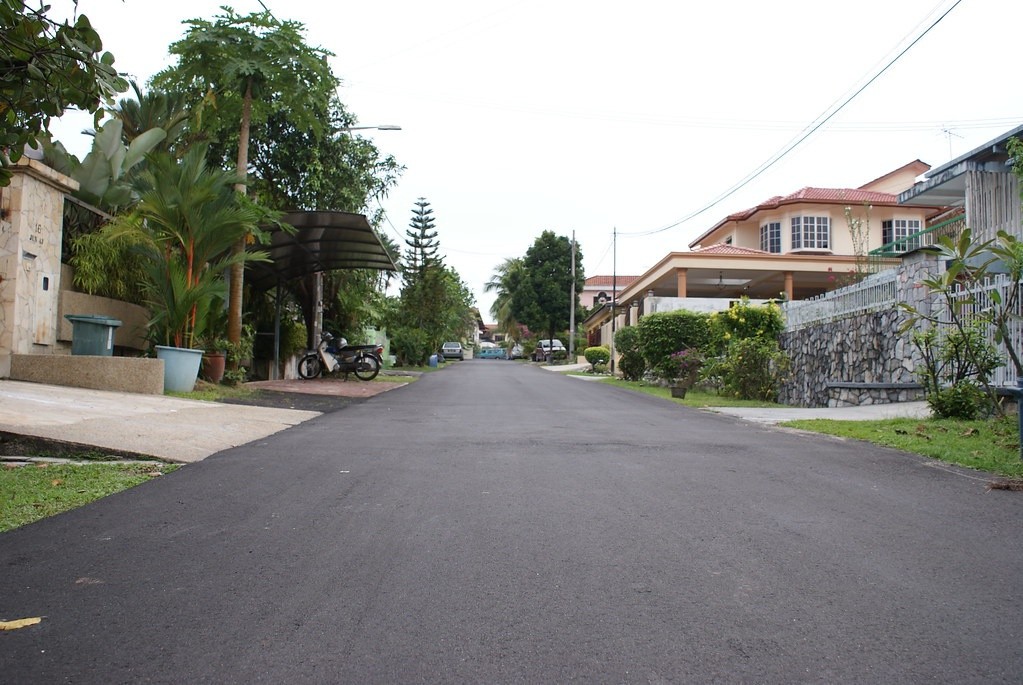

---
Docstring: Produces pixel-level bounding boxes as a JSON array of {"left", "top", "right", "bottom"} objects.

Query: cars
[
  {"left": 441, "top": 342, "right": 465, "bottom": 361},
  {"left": 473, "top": 348, "right": 507, "bottom": 360},
  {"left": 536, "top": 339, "right": 566, "bottom": 361},
  {"left": 507, "top": 345, "right": 524, "bottom": 360}
]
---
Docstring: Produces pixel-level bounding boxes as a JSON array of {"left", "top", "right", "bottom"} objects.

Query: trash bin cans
[
  {"left": 64, "top": 314, "right": 122, "bottom": 357},
  {"left": 546, "top": 354, "right": 554, "bottom": 365},
  {"left": 529, "top": 353, "right": 537, "bottom": 361}
]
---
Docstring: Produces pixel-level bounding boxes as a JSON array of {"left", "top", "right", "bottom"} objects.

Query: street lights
[{"left": 312, "top": 125, "right": 402, "bottom": 373}]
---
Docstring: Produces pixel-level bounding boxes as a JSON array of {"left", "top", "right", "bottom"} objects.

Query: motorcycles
[{"left": 297, "top": 330, "right": 384, "bottom": 382}]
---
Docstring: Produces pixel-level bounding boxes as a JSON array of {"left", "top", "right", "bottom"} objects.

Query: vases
[{"left": 671, "top": 387, "right": 687, "bottom": 399}]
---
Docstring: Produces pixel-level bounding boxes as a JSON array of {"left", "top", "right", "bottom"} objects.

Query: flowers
[{"left": 662, "top": 349, "right": 701, "bottom": 388}]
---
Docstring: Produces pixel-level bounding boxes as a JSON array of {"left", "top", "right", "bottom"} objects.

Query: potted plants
[{"left": 198, "top": 335, "right": 235, "bottom": 384}]
